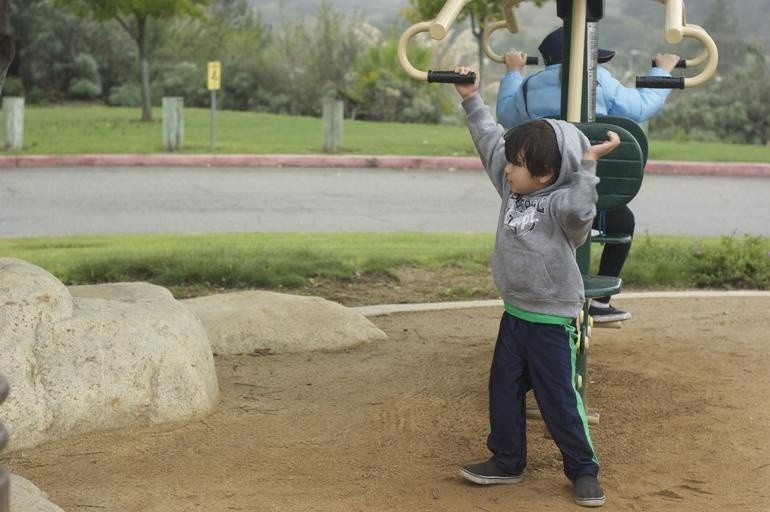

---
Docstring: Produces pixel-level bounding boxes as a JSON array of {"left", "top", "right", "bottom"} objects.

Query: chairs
[{"left": 526, "top": 115, "right": 648, "bottom": 425}]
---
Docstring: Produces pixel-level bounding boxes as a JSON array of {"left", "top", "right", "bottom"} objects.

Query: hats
[{"left": 539, "top": 26, "right": 616, "bottom": 65}]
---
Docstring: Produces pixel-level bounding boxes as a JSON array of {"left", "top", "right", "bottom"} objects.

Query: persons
[
  {"left": 495, "top": 27, "right": 679, "bottom": 322},
  {"left": 449, "top": 65, "right": 620, "bottom": 506}
]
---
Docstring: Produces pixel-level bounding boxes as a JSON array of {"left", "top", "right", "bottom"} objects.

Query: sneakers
[
  {"left": 459, "top": 461, "right": 523, "bottom": 485},
  {"left": 589, "top": 305, "right": 631, "bottom": 322},
  {"left": 571, "top": 477, "right": 606, "bottom": 507}
]
